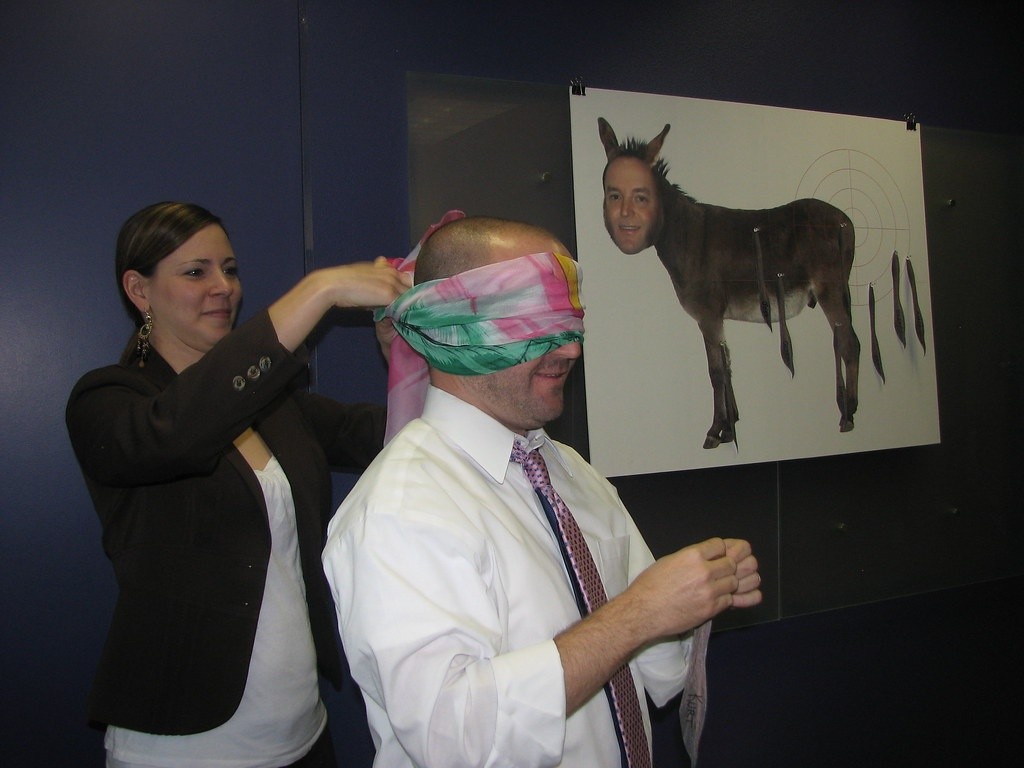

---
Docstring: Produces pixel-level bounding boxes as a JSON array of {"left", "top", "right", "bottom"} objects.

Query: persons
[
  {"left": 65, "top": 201, "right": 414, "bottom": 768},
  {"left": 603, "top": 153, "right": 658, "bottom": 255},
  {"left": 321, "top": 215, "right": 761, "bottom": 767}
]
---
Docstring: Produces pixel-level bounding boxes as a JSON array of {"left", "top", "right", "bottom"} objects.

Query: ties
[{"left": 509, "top": 440, "right": 652, "bottom": 768}]
